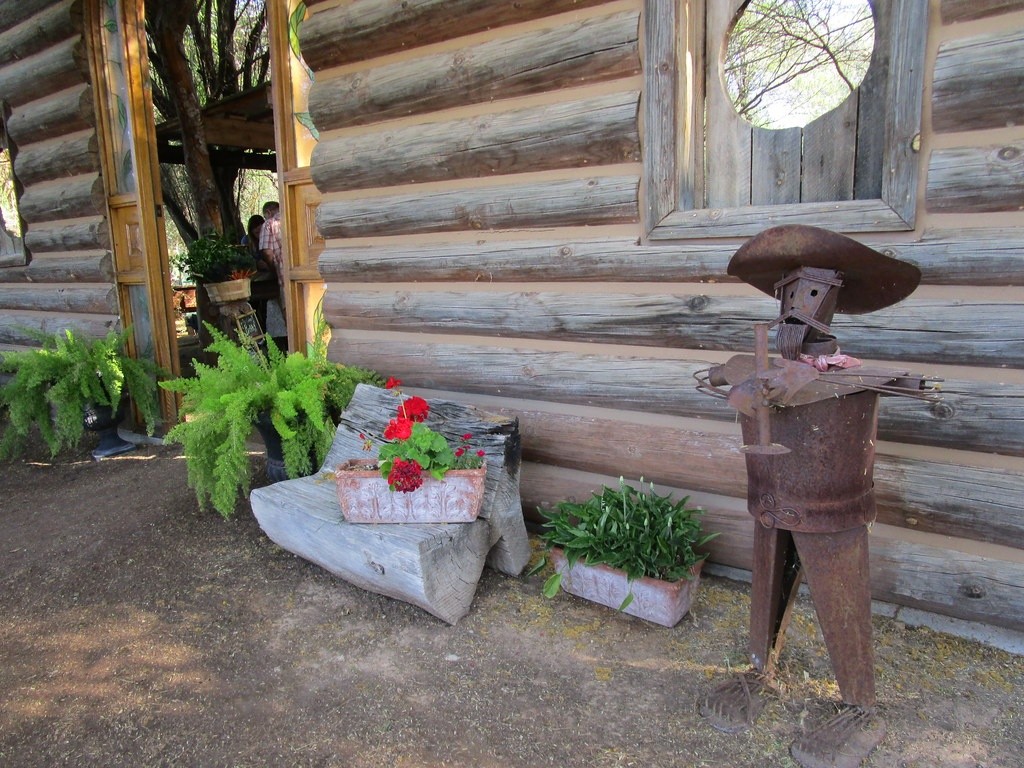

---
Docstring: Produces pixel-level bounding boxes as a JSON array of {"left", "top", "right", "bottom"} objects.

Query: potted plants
[
  {"left": 158, "top": 290, "right": 388, "bottom": 523},
  {"left": 0, "top": 321, "right": 179, "bottom": 458},
  {"left": 175, "top": 231, "right": 256, "bottom": 303}
]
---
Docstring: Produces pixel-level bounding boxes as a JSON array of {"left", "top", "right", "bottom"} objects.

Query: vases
[
  {"left": 538, "top": 545, "right": 708, "bottom": 628},
  {"left": 335, "top": 459, "right": 488, "bottom": 524}
]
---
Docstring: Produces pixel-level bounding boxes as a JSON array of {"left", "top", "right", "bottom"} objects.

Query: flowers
[
  {"left": 536, "top": 473, "right": 721, "bottom": 581},
  {"left": 360, "top": 376, "right": 485, "bottom": 493}
]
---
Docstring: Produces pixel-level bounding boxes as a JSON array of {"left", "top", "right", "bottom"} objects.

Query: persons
[{"left": 241, "top": 201, "right": 287, "bottom": 327}]
